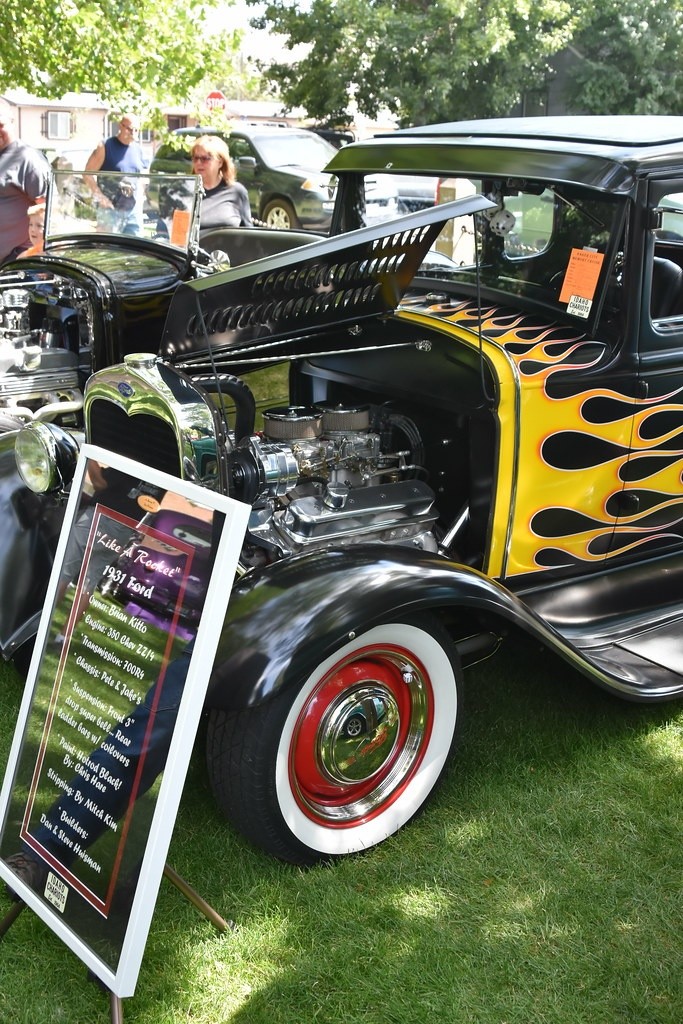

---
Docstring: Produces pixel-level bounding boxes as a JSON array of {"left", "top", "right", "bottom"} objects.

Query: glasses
[
  {"left": 121, "top": 122, "right": 138, "bottom": 133},
  {"left": 191, "top": 155, "right": 215, "bottom": 163}
]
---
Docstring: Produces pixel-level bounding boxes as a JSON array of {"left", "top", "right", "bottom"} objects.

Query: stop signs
[{"left": 205, "top": 90, "right": 228, "bottom": 114}]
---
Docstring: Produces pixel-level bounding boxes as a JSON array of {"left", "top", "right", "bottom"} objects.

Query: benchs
[{"left": 198, "top": 227, "right": 335, "bottom": 269}]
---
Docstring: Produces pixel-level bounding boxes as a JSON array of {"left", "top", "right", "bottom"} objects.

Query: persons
[
  {"left": 8, "top": 459, "right": 196, "bottom": 887},
  {"left": 156, "top": 135, "right": 253, "bottom": 243},
  {"left": 0, "top": 98, "right": 60, "bottom": 268},
  {"left": 17, "top": 203, "right": 46, "bottom": 259},
  {"left": 83, "top": 112, "right": 147, "bottom": 238}
]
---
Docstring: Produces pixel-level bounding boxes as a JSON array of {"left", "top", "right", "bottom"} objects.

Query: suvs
[
  {"left": 0, "top": 115, "right": 683, "bottom": 868},
  {"left": 143, "top": 124, "right": 399, "bottom": 233}
]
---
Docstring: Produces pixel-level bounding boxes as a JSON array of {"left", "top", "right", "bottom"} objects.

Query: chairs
[{"left": 649, "top": 256, "right": 683, "bottom": 321}]
